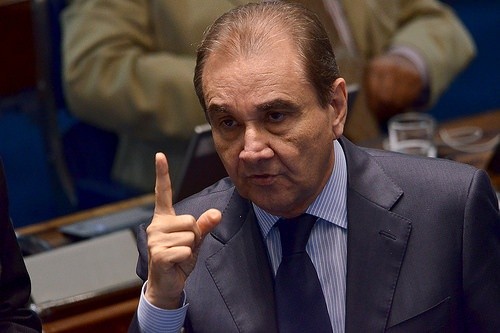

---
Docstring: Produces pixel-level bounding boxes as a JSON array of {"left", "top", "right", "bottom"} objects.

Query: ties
[{"left": 274, "top": 213, "right": 333, "bottom": 333}]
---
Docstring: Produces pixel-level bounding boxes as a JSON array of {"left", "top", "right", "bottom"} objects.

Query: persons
[
  {"left": 129, "top": 2, "right": 500, "bottom": 333},
  {"left": 61, "top": 0, "right": 476, "bottom": 192}
]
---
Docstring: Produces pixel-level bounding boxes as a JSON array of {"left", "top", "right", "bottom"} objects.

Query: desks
[{"left": 13, "top": 110, "right": 500, "bottom": 333}]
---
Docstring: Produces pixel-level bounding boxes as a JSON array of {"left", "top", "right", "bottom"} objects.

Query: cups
[{"left": 387, "top": 113, "right": 435, "bottom": 158}]
